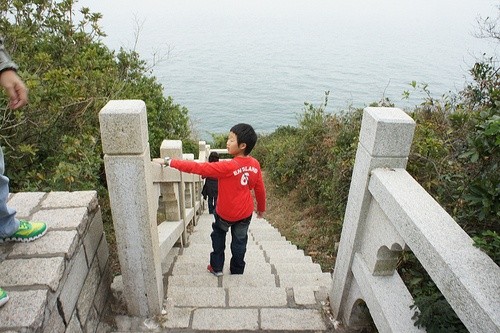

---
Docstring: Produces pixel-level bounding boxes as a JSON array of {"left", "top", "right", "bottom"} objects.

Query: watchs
[{"left": 164, "top": 157, "right": 170, "bottom": 166}]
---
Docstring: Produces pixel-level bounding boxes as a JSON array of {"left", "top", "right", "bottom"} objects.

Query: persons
[
  {"left": 0, "top": 33, "right": 47, "bottom": 309},
  {"left": 152, "top": 123, "right": 266, "bottom": 277},
  {"left": 201, "top": 152, "right": 219, "bottom": 214}
]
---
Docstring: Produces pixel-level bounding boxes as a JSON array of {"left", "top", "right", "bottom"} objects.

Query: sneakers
[
  {"left": 0, "top": 219, "right": 48, "bottom": 243},
  {"left": 207, "top": 265, "right": 224, "bottom": 277},
  {"left": 0, "top": 287, "right": 9, "bottom": 307}
]
